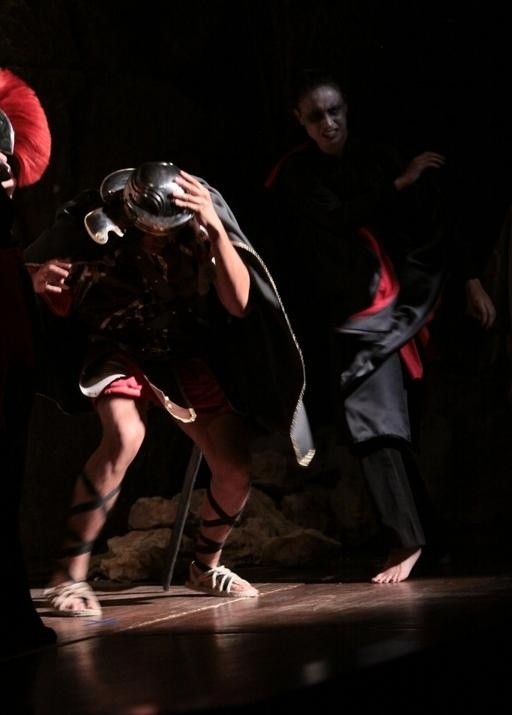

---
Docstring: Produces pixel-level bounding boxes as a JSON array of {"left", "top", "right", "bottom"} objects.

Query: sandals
[
  {"left": 44, "top": 577, "right": 103, "bottom": 618},
  {"left": 187, "top": 559, "right": 259, "bottom": 597}
]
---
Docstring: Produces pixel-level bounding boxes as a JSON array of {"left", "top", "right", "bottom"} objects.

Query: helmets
[{"left": 85, "top": 160, "right": 195, "bottom": 244}]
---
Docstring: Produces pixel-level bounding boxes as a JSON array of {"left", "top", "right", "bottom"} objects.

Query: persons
[
  {"left": 29, "top": 162, "right": 315, "bottom": 619},
  {"left": 269, "top": 74, "right": 497, "bottom": 585},
  {"left": 1, "top": 65, "right": 57, "bottom": 654}
]
